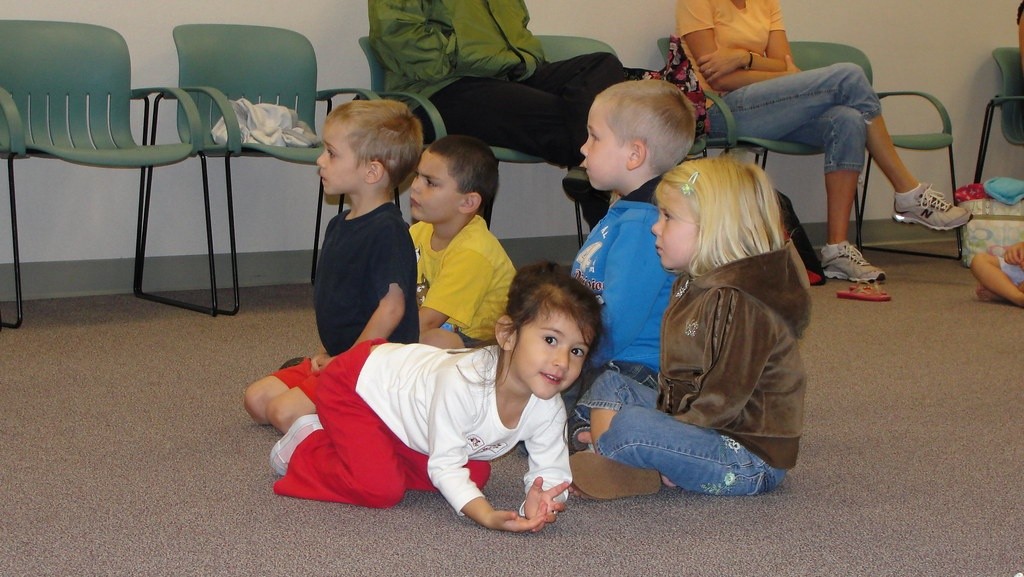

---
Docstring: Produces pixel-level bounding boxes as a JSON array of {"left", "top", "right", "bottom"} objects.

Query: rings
[{"left": 709, "top": 69, "right": 712, "bottom": 72}]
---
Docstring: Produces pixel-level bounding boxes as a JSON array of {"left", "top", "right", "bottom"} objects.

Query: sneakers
[
  {"left": 269, "top": 413, "right": 323, "bottom": 477},
  {"left": 888, "top": 182, "right": 973, "bottom": 232},
  {"left": 821, "top": 240, "right": 887, "bottom": 284}
]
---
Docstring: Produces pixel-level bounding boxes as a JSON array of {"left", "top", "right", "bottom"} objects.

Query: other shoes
[{"left": 568, "top": 451, "right": 662, "bottom": 499}]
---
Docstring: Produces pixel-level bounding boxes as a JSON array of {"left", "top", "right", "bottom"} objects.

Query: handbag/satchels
[{"left": 623, "top": 32, "right": 711, "bottom": 137}]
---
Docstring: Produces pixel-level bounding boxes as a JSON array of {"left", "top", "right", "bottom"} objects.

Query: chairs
[
  {"left": 788, "top": 41, "right": 963, "bottom": 261},
  {"left": 140, "top": 23, "right": 382, "bottom": 316},
  {"left": 967, "top": 45, "right": 1024, "bottom": 183},
  {"left": 0, "top": 19, "right": 217, "bottom": 329},
  {"left": 657, "top": 36, "right": 862, "bottom": 254},
  {"left": 357, "top": 35, "right": 584, "bottom": 250},
  {"left": 534, "top": 36, "right": 708, "bottom": 158}
]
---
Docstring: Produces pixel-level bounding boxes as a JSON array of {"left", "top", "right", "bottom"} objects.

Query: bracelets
[{"left": 745, "top": 52, "right": 753, "bottom": 70}]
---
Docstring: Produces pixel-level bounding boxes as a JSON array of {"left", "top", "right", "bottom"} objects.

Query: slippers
[{"left": 836, "top": 280, "right": 891, "bottom": 302}]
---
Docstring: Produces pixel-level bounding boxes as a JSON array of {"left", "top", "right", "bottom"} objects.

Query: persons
[
  {"left": 243, "top": 100, "right": 424, "bottom": 432},
  {"left": 269, "top": 261, "right": 604, "bottom": 534},
  {"left": 518, "top": 79, "right": 697, "bottom": 456},
  {"left": 674, "top": 0, "right": 972, "bottom": 283},
  {"left": 365, "top": 0, "right": 660, "bottom": 231},
  {"left": 568, "top": 157, "right": 813, "bottom": 500},
  {"left": 409, "top": 136, "right": 517, "bottom": 350},
  {"left": 971, "top": 241, "right": 1024, "bottom": 308}
]
